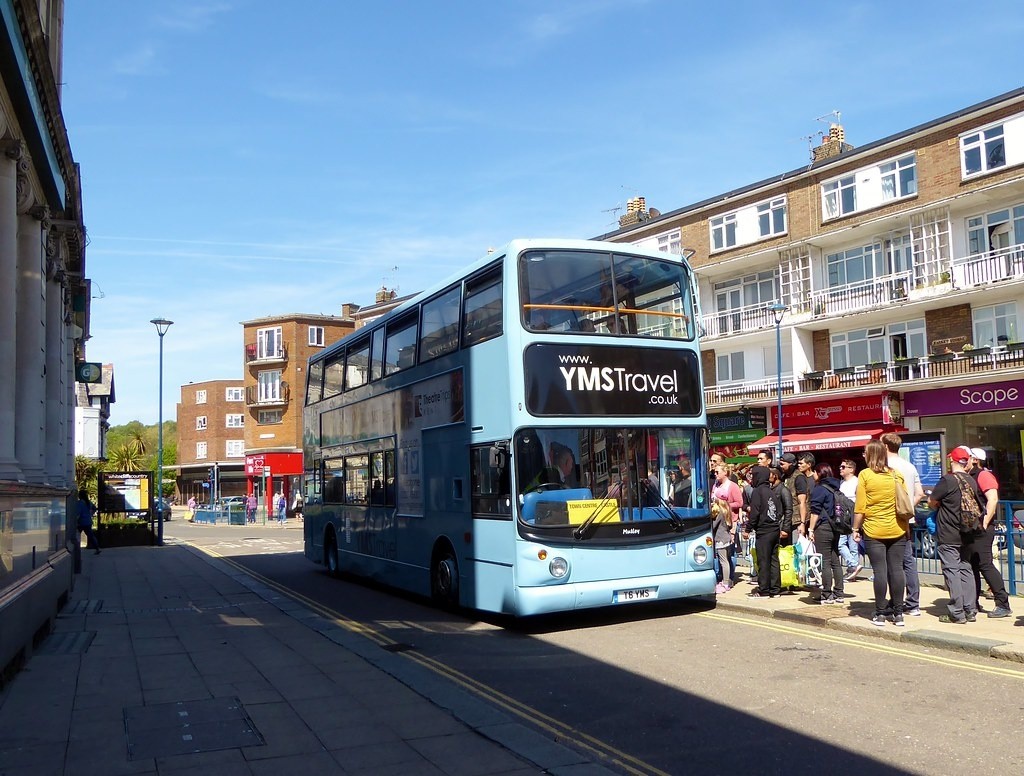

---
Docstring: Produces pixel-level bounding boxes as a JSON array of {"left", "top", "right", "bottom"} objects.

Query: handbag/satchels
[
  {"left": 925, "top": 508, "right": 938, "bottom": 535},
  {"left": 794, "top": 533, "right": 814, "bottom": 578},
  {"left": 184, "top": 511, "right": 193, "bottom": 520},
  {"left": 750, "top": 543, "right": 799, "bottom": 588},
  {"left": 805, "top": 540, "right": 822, "bottom": 587},
  {"left": 894, "top": 470, "right": 914, "bottom": 521}
]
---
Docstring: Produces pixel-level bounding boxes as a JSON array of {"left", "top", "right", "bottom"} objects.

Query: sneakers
[
  {"left": 903, "top": 608, "right": 921, "bottom": 616},
  {"left": 893, "top": 614, "right": 904, "bottom": 625},
  {"left": 870, "top": 614, "right": 886, "bottom": 626},
  {"left": 965, "top": 611, "right": 976, "bottom": 622},
  {"left": 939, "top": 614, "right": 967, "bottom": 624},
  {"left": 988, "top": 606, "right": 1013, "bottom": 618},
  {"left": 715, "top": 565, "right": 863, "bottom": 603}
]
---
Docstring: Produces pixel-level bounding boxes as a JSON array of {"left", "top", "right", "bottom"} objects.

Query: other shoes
[{"left": 94, "top": 548, "right": 102, "bottom": 554}]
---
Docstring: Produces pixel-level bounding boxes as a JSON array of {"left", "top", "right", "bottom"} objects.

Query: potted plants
[
  {"left": 929, "top": 346, "right": 955, "bottom": 361},
  {"left": 865, "top": 361, "right": 887, "bottom": 370},
  {"left": 962, "top": 342, "right": 991, "bottom": 356},
  {"left": 1006, "top": 323, "right": 1024, "bottom": 351},
  {"left": 893, "top": 353, "right": 918, "bottom": 365},
  {"left": 909, "top": 272, "right": 952, "bottom": 300},
  {"left": 803, "top": 370, "right": 824, "bottom": 379}
]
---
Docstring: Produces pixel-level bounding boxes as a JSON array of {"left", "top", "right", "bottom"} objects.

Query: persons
[
  {"left": 606, "top": 315, "right": 627, "bottom": 334},
  {"left": 78, "top": 490, "right": 102, "bottom": 555},
  {"left": 808, "top": 462, "right": 844, "bottom": 603},
  {"left": 927, "top": 445, "right": 988, "bottom": 624},
  {"left": 188, "top": 495, "right": 196, "bottom": 522},
  {"left": 521, "top": 446, "right": 573, "bottom": 494},
  {"left": 838, "top": 459, "right": 863, "bottom": 583},
  {"left": 579, "top": 319, "right": 597, "bottom": 333},
  {"left": 966, "top": 448, "right": 1013, "bottom": 618},
  {"left": 243, "top": 493, "right": 256, "bottom": 524},
  {"left": 276, "top": 494, "right": 287, "bottom": 524},
  {"left": 852, "top": 439, "right": 908, "bottom": 625},
  {"left": 880, "top": 433, "right": 924, "bottom": 616},
  {"left": 608, "top": 449, "right": 820, "bottom": 599},
  {"left": 292, "top": 498, "right": 303, "bottom": 522}
]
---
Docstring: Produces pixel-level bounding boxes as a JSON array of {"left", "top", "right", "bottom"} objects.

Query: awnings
[{"left": 747, "top": 423, "right": 908, "bottom": 456}]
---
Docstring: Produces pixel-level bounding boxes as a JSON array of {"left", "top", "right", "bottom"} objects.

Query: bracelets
[
  {"left": 852, "top": 528, "right": 859, "bottom": 532},
  {"left": 808, "top": 528, "right": 814, "bottom": 532},
  {"left": 801, "top": 522, "right": 805, "bottom": 524}
]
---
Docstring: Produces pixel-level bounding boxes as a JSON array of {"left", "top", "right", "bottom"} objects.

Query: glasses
[
  {"left": 710, "top": 460, "right": 717, "bottom": 463},
  {"left": 862, "top": 453, "right": 866, "bottom": 457},
  {"left": 713, "top": 471, "right": 719, "bottom": 475},
  {"left": 838, "top": 465, "right": 852, "bottom": 470}
]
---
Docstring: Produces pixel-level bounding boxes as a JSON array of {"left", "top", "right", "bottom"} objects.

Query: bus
[{"left": 301, "top": 237, "right": 715, "bottom": 618}]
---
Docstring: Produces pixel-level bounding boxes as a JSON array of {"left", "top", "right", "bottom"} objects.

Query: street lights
[
  {"left": 149, "top": 316, "right": 174, "bottom": 545},
  {"left": 765, "top": 303, "right": 789, "bottom": 456}
]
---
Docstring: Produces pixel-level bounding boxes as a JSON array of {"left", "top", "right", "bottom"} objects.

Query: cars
[
  {"left": 195, "top": 495, "right": 249, "bottom": 516},
  {"left": 126, "top": 498, "right": 171, "bottom": 522}
]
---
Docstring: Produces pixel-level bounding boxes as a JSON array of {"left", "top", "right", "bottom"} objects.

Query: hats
[
  {"left": 780, "top": 453, "right": 797, "bottom": 465},
  {"left": 957, "top": 445, "right": 972, "bottom": 456},
  {"left": 970, "top": 448, "right": 986, "bottom": 461},
  {"left": 947, "top": 447, "right": 970, "bottom": 464}
]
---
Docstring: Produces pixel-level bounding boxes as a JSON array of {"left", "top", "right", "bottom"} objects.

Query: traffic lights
[{"left": 207, "top": 468, "right": 213, "bottom": 480}]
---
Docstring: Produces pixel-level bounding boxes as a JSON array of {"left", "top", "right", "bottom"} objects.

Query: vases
[{"left": 834, "top": 367, "right": 854, "bottom": 374}]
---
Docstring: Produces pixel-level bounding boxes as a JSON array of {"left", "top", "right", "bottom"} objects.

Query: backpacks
[
  {"left": 954, "top": 471, "right": 983, "bottom": 534},
  {"left": 819, "top": 483, "right": 855, "bottom": 535}
]
